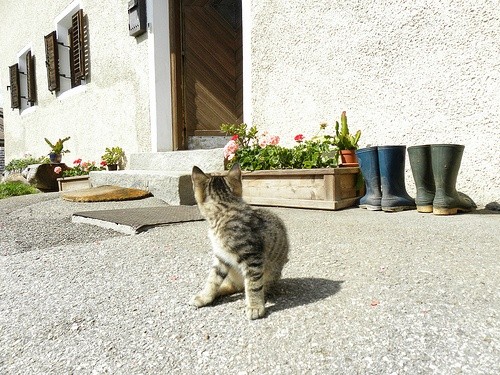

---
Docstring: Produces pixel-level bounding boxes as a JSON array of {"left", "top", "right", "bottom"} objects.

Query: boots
[
  {"left": 356, "top": 146, "right": 382, "bottom": 211},
  {"left": 406, "top": 144, "right": 436, "bottom": 213},
  {"left": 377, "top": 144, "right": 418, "bottom": 212},
  {"left": 428, "top": 144, "right": 472, "bottom": 215}
]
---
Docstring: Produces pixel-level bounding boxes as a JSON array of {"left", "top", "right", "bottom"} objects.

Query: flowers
[
  {"left": 54, "top": 159, "right": 107, "bottom": 180},
  {"left": 220, "top": 123, "right": 339, "bottom": 170}
]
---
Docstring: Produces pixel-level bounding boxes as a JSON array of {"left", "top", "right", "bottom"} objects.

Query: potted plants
[
  {"left": 44, "top": 137, "right": 71, "bottom": 163},
  {"left": 101, "top": 146, "right": 125, "bottom": 171},
  {"left": 322, "top": 111, "right": 361, "bottom": 165}
]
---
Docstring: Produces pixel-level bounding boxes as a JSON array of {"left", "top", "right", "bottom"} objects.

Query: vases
[
  {"left": 57, "top": 176, "right": 91, "bottom": 191},
  {"left": 212, "top": 167, "right": 365, "bottom": 210}
]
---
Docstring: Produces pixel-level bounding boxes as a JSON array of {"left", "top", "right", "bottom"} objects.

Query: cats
[{"left": 191, "top": 163, "right": 289, "bottom": 321}]
